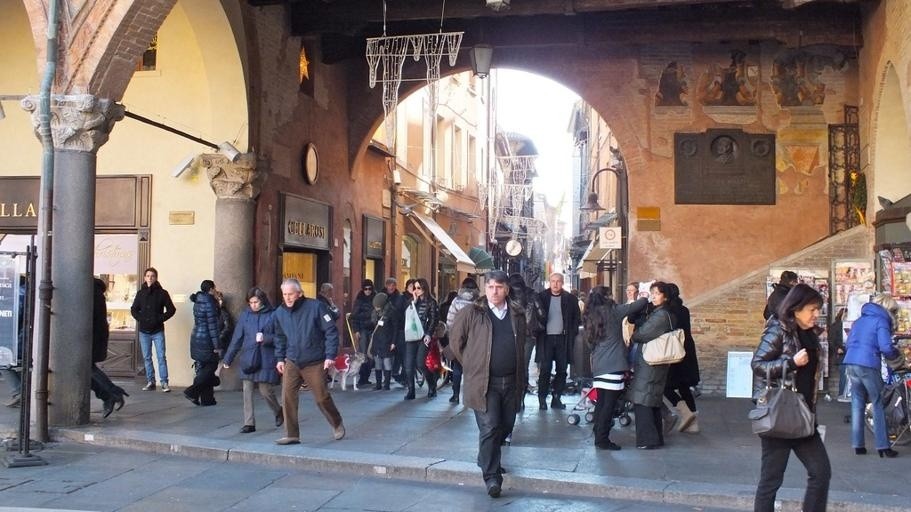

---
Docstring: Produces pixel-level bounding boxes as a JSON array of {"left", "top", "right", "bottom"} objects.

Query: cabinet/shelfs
[
  {"left": 837, "top": 290, "right": 872, "bottom": 404},
  {"left": 766, "top": 265, "right": 829, "bottom": 391},
  {"left": 832, "top": 258, "right": 875, "bottom": 324},
  {"left": 872, "top": 249, "right": 911, "bottom": 384}
]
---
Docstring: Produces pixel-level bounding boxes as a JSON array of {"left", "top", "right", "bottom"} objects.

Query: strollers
[
  {"left": 863, "top": 337, "right": 911, "bottom": 449},
  {"left": 565, "top": 366, "right": 638, "bottom": 426}
]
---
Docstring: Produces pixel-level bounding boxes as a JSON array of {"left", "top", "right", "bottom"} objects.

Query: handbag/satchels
[
  {"left": 642, "top": 312, "right": 686, "bottom": 366},
  {"left": 749, "top": 360, "right": 815, "bottom": 439},
  {"left": 881, "top": 382, "right": 907, "bottom": 425},
  {"left": 240, "top": 344, "right": 261, "bottom": 374}
]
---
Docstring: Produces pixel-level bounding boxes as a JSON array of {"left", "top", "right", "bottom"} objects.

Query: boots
[
  {"left": 661, "top": 400, "right": 698, "bottom": 433},
  {"left": 375, "top": 370, "right": 391, "bottom": 390}
]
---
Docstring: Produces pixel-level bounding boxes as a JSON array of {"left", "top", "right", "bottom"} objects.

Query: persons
[
  {"left": 764, "top": 271, "right": 798, "bottom": 320},
  {"left": 829, "top": 308, "right": 853, "bottom": 402},
  {"left": 0, "top": 276, "right": 130, "bottom": 418},
  {"left": 510, "top": 274, "right": 702, "bottom": 450},
  {"left": 202, "top": 290, "right": 233, "bottom": 386},
  {"left": 320, "top": 277, "right": 481, "bottom": 404},
  {"left": 274, "top": 278, "right": 346, "bottom": 445},
  {"left": 449, "top": 270, "right": 527, "bottom": 498},
  {"left": 223, "top": 288, "right": 285, "bottom": 433},
  {"left": 751, "top": 284, "right": 831, "bottom": 512},
  {"left": 132, "top": 268, "right": 176, "bottom": 392},
  {"left": 844, "top": 293, "right": 911, "bottom": 457},
  {"left": 184, "top": 280, "right": 225, "bottom": 405}
]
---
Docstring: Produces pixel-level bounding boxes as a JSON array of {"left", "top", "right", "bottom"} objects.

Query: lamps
[
  {"left": 469, "top": 21, "right": 494, "bottom": 79},
  {"left": 481, "top": 0, "right": 512, "bottom": 12},
  {"left": 503, "top": 240, "right": 522, "bottom": 261}
]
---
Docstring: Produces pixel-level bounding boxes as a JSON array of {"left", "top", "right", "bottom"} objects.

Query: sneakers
[
  {"left": 6, "top": 394, "right": 22, "bottom": 407},
  {"left": 142, "top": 383, "right": 171, "bottom": 392}
]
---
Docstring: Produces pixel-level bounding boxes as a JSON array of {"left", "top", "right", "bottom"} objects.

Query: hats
[
  {"left": 373, "top": 292, "right": 387, "bottom": 307},
  {"left": 362, "top": 279, "right": 372, "bottom": 287}
]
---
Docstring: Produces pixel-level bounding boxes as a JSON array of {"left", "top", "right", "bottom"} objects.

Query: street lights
[{"left": 580, "top": 166, "right": 626, "bottom": 327}]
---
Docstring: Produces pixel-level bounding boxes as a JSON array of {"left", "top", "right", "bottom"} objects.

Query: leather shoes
[
  {"left": 404, "top": 388, "right": 459, "bottom": 403},
  {"left": 334, "top": 419, "right": 345, "bottom": 439},
  {"left": 596, "top": 441, "right": 657, "bottom": 449},
  {"left": 540, "top": 400, "right": 565, "bottom": 409},
  {"left": 489, "top": 468, "right": 505, "bottom": 497},
  {"left": 240, "top": 425, "right": 255, "bottom": 432},
  {"left": 276, "top": 437, "right": 299, "bottom": 444},
  {"left": 186, "top": 396, "right": 216, "bottom": 406},
  {"left": 276, "top": 406, "right": 284, "bottom": 426}
]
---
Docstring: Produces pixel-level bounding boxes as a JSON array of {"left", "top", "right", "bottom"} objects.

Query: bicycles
[{"left": 415, "top": 341, "right": 451, "bottom": 390}]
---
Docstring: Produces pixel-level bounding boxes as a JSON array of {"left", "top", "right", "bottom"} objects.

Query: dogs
[{"left": 328, "top": 352, "right": 368, "bottom": 391}]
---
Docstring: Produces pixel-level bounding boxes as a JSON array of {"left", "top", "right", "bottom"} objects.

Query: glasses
[
  {"left": 364, "top": 288, "right": 372, "bottom": 291},
  {"left": 414, "top": 287, "right": 421, "bottom": 290}
]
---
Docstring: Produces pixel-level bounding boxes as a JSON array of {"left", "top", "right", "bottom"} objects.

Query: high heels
[
  {"left": 856, "top": 448, "right": 898, "bottom": 457},
  {"left": 103, "top": 389, "right": 128, "bottom": 417}
]
